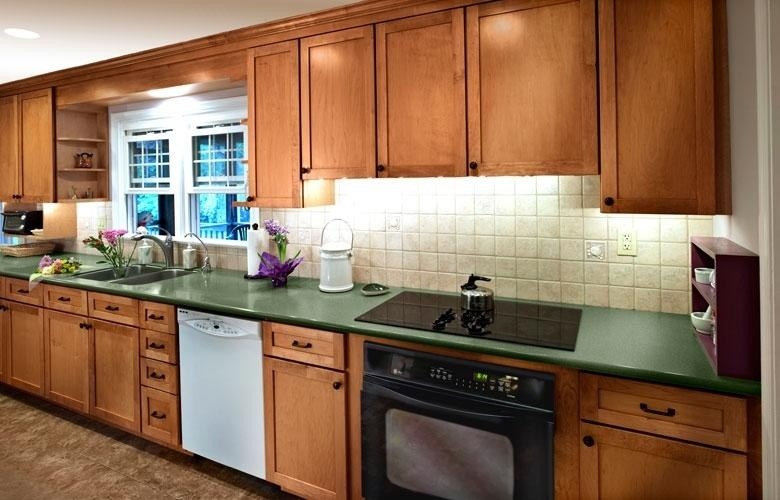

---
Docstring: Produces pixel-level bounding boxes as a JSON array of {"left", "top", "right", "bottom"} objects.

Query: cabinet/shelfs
[
  {"left": 56, "top": 107, "right": 109, "bottom": 202},
  {"left": 466, "top": 0, "right": 596, "bottom": 178},
  {"left": 262, "top": 319, "right": 347, "bottom": 500},
  {"left": 598, "top": 1, "right": 727, "bottom": 214},
  {"left": 301, "top": 13, "right": 376, "bottom": 179},
  {"left": 1, "top": 92, "right": 21, "bottom": 203},
  {"left": 19, "top": 87, "right": 55, "bottom": 205},
  {"left": 376, "top": 1, "right": 468, "bottom": 178},
  {"left": 88, "top": 290, "right": 141, "bottom": 435},
  {"left": 139, "top": 301, "right": 181, "bottom": 453},
  {"left": 578, "top": 369, "right": 751, "bottom": 500},
  {"left": 248, "top": 38, "right": 336, "bottom": 207},
  {"left": 43, "top": 284, "right": 87, "bottom": 415},
  {"left": 690, "top": 235, "right": 760, "bottom": 378},
  {"left": 6, "top": 278, "right": 44, "bottom": 398},
  {"left": 1, "top": 274, "right": 6, "bottom": 383}
]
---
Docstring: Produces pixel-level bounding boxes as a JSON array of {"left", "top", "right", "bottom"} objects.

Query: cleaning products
[
  {"left": 183, "top": 241, "right": 197, "bottom": 270},
  {"left": 183, "top": 237, "right": 198, "bottom": 269}
]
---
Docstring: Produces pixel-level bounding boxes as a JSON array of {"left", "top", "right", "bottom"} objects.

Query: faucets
[
  {"left": 131, "top": 226, "right": 173, "bottom": 267},
  {"left": 183, "top": 232, "right": 212, "bottom": 272}
]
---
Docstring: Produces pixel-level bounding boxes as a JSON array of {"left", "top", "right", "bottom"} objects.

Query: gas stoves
[{"left": 355, "top": 291, "right": 582, "bottom": 498}]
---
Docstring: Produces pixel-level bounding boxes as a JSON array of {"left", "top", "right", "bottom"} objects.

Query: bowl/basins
[
  {"left": 690, "top": 312, "right": 712, "bottom": 335},
  {"left": 695, "top": 267, "right": 712, "bottom": 284}
]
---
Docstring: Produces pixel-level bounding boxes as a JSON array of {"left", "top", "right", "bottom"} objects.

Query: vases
[
  {"left": 272, "top": 276, "right": 288, "bottom": 288},
  {"left": 113, "top": 267, "right": 129, "bottom": 279}
]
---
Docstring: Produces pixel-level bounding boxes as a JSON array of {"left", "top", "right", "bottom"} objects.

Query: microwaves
[{"left": 1, "top": 210, "right": 43, "bottom": 235}]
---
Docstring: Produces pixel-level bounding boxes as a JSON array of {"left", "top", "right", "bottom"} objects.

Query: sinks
[
  {"left": 73, "top": 262, "right": 162, "bottom": 282},
  {"left": 108, "top": 268, "right": 197, "bottom": 285}
]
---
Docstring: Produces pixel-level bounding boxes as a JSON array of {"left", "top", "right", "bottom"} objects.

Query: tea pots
[{"left": 460, "top": 273, "right": 494, "bottom": 310}]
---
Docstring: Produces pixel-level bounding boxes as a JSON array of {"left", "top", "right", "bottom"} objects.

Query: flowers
[
  {"left": 82, "top": 226, "right": 149, "bottom": 267},
  {"left": 257, "top": 218, "right": 304, "bottom": 276},
  {"left": 37, "top": 255, "right": 80, "bottom": 275}
]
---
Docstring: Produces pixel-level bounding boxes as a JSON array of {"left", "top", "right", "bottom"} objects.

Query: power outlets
[{"left": 618, "top": 228, "right": 636, "bottom": 255}]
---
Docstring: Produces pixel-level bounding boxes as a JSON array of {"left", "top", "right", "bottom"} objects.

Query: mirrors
[{"left": 112, "top": 98, "right": 249, "bottom": 240}]
